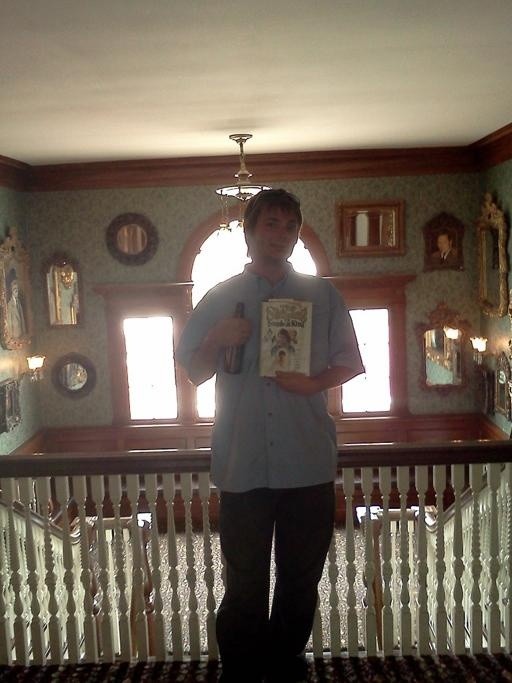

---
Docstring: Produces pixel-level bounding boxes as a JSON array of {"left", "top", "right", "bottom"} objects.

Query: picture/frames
[
  {"left": 422, "top": 208, "right": 464, "bottom": 271},
  {"left": 0, "top": 379, "right": 21, "bottom": 432},
  {"left": 0, "top": 224, "right": 35, "bottom": 350},
  {"left": 471, "top": 191, "right": 507, "bottom": 318}
]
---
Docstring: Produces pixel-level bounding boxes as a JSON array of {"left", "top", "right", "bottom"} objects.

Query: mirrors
[
  {"left": 49, "top": 353, "right": 96, "bottom": 398},
  {"left": 105, "top": 213, "right": 159, "bottom": 268},
  {"left": 492, "top": 350, "right": 510, "bottom": 419},
  {"left": 336, "top": 197, "right": 406, "bottom": 256},
  {"left": 415, "top": 302, "right": 469, "bottom": 395},
  {"left": 41, "top": 249, "right": 83, "bottom": 329}
]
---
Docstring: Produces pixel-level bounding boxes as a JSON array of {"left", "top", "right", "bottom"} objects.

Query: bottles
[{"left": 224, "top": 303, "right": 245, "bottom": 374}]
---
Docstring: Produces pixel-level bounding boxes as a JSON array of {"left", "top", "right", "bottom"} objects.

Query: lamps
[{"left": 214, "top": 133, "right": 270, "bottom": 231}]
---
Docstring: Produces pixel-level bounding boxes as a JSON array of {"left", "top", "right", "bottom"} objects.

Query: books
[{"left": 260, "top": 299, "right": 313, "bottom": 378}]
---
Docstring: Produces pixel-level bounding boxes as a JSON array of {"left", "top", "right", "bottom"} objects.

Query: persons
[
  {"left": 271, "top": 327, "right": 298, "bottom": 366},
  {"left": 7, "top": 280, "right": 26, "bottom": 337},
  {"left": 433, "top": 232, "right": 458, "bottom": 268},
  {"left": 175, "top": 192, "right": 365, "bottom": 683}
]
